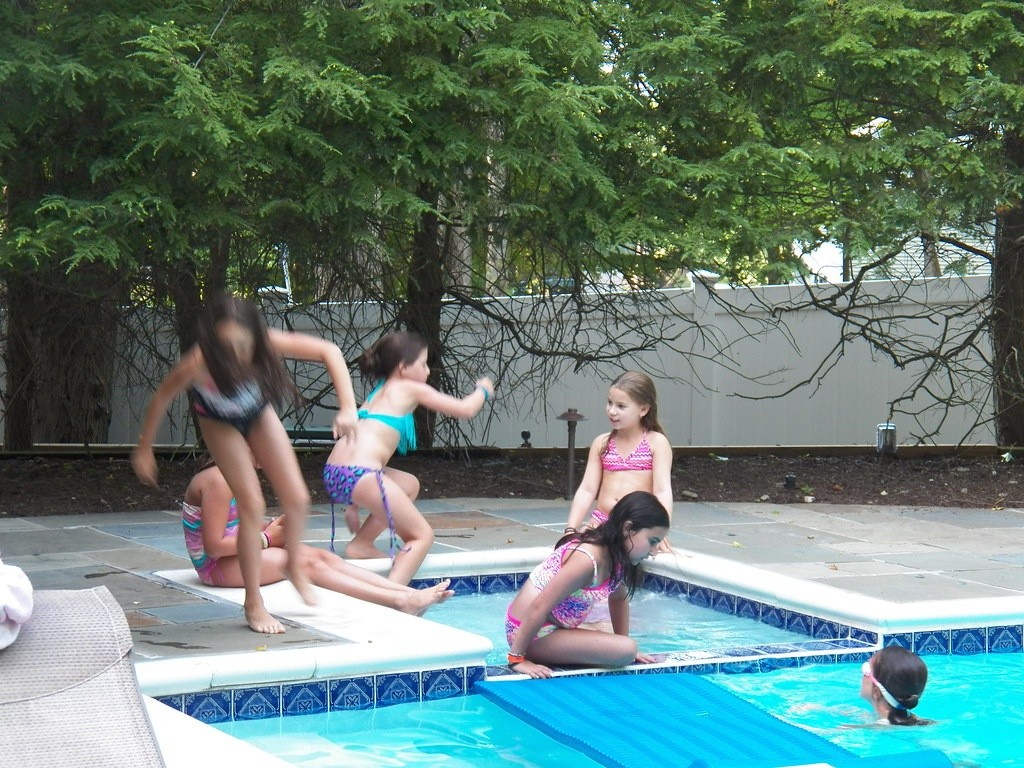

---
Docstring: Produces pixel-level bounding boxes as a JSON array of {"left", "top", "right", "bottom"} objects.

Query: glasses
[{"left": 862, "top": 661, "right": 878, "bottom": 686}]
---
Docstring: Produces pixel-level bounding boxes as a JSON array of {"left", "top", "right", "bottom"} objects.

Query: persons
[
  {"left": 183, "top": 446, "right": 455, "bottom": 617},
  {"left": 555, "top": 369, "right": 681, "bottom": 555},
  {"left": 134, "top": 298, "right": 358, "bottom": 633},
  {"left": 505, "top": 490, "right": 670, "bottom": 679},
  {"left": 324, "top": 333, "right": 493, "bottom": 585},
  {"left": 861, "top": 646, "right": 928, "bottom": 724}
]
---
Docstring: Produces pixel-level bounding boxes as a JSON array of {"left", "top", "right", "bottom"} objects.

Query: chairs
[{"left": 0, "top": 584, "right": 167, "bottom": 768}]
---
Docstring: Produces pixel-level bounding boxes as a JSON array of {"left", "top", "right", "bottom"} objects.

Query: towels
[{"left": 0, "top": 557, "right": 37, "bottom": 654}]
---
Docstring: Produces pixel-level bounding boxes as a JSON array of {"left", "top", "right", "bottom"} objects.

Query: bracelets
[
  {"left": 508, "top": 652, "right": 526, "bottom": 663},
  {"left": 565, "top": 527, "right": 577, "bottom": 533},
  {"left": 259, "top": 531, "right": 273, "bottom": 549},
  {"left": 477, "top": 384, "right": 489, "bottom": 400}
]
